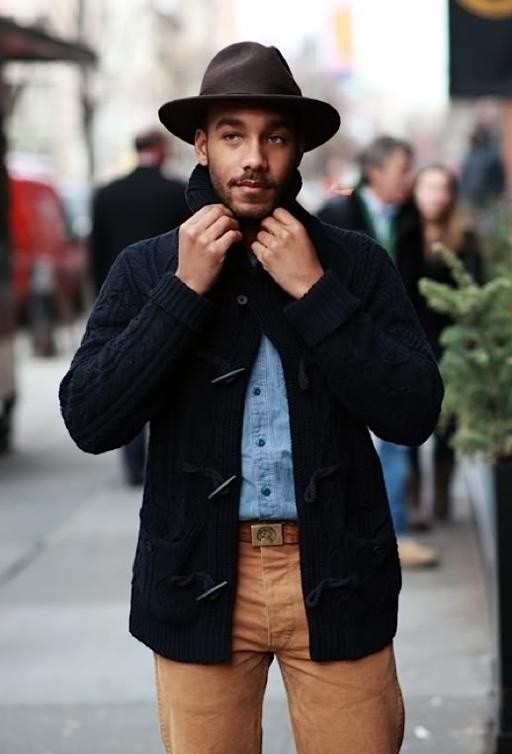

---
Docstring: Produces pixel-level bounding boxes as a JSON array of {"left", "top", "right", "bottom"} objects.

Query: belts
[{"left": 239, "top": 522, "right": 299, "bottom": 548}]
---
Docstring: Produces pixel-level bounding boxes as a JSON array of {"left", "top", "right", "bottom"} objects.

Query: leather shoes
[{"left": 396, "top": 534, "right": 438, "bottom": 565}]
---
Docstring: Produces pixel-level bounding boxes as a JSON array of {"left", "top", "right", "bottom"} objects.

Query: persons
[
  {"left": 58, "top": 40, "right": 443, "bottom": 754},
  {"left": 91, "top": 127, "right": 189, "bottom": 486},
  {"left": 0, "top": 131, "right": 19, "bottom": 458},
  {"left": 313, "top": 135, "right": 443, "bottom": 571},
  {"left": 406, "top": 160, "right": 482, "bottom": 531}
]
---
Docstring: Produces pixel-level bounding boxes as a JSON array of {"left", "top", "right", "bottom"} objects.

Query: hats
[{"left": 158, "top": 41, "right": 340, "bottom": 151}]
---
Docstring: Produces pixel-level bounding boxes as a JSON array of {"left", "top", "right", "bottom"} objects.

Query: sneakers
[{"left": 404, "top": 505, "right": 430, "bottom": 533}]
